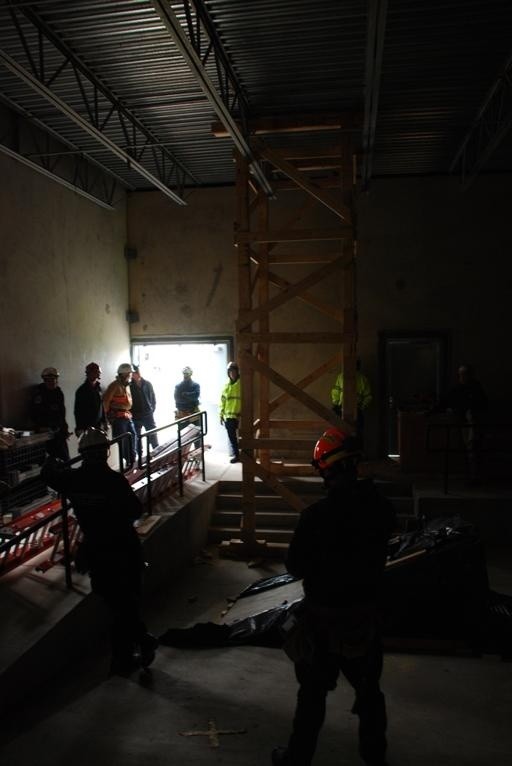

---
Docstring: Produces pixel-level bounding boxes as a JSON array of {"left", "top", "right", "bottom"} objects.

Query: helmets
[
  {"left": 311, "top": 428, "right": 354, "bottom": 470},
  {"left": 183, "top": 362, "right": 240, "bottom": 377},
  {"left": 77, "top": 427, "right": 111, "bottom": 458},
  {"left": 41, "top": 361, "right": 143, "bottom": 380}
]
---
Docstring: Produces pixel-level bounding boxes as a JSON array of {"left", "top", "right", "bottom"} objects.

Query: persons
[
  {"left": 38, "top": 424, "right": 159, "bottom": 684},
  {"left": 174, "top": 365, "right": 202, "bottom": 463},
  {"left": 331, "top": 362, "right": 375, "bottom": 475},
  {"left": 74, "top": 362, "right": 110, "bottom": 437},
  {"left": 219, "top": 361, "right": 243, "bottom": 464},
  {"left": 102, "top": 363, "right": 137, "bottom": 466},
  {"left": 127, "top": 364, "right": 159, "bottom": 456},
  {"left": 268, "top": 426, "right": 399, "bottom": 766},
  {"left": 29, "top": 367, "right": 72, "bottom": 470},
  {"left": 445, "top": 364, "right": 490, "bottom": 477}
]
